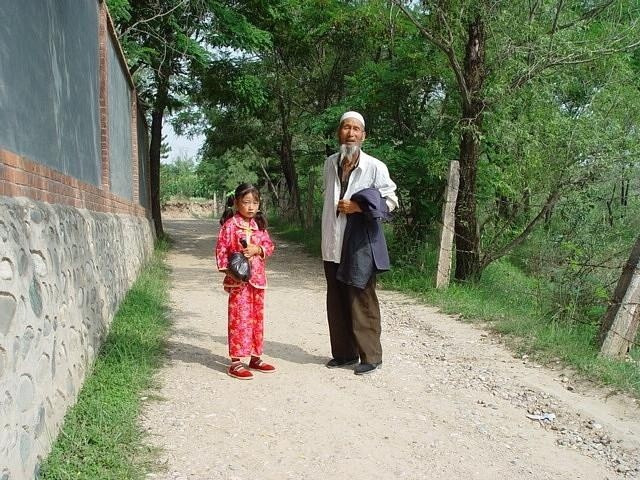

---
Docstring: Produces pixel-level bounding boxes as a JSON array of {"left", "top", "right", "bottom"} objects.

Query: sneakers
[
  {"left": 326, "top": 355, "right": 359, "bottom": 369},
  {"left": 227, "top": 360, "right": 254, "bottom": 380},
  {"left": 248, "top": 356, "right": 276, "bottom": 373},
  {"left": 353, "top": 360, "right": 383, "bottom": 375}
]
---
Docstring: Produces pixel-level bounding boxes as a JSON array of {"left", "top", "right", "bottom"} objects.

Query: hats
[{"left": 339, "top": 110, "right": 365, "bottom": 128}]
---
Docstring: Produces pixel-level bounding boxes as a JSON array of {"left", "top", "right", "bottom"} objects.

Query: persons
[
  {"left": 215, "top": 181, "right": 277, "bottom": 380},
  {"left": 319, "top": 110, "right": 400, "bottom": 374}
]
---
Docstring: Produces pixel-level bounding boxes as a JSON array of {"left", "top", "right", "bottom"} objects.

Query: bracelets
[{"left": 258, "top": 246, "right": 262, "bottom": 255}]
